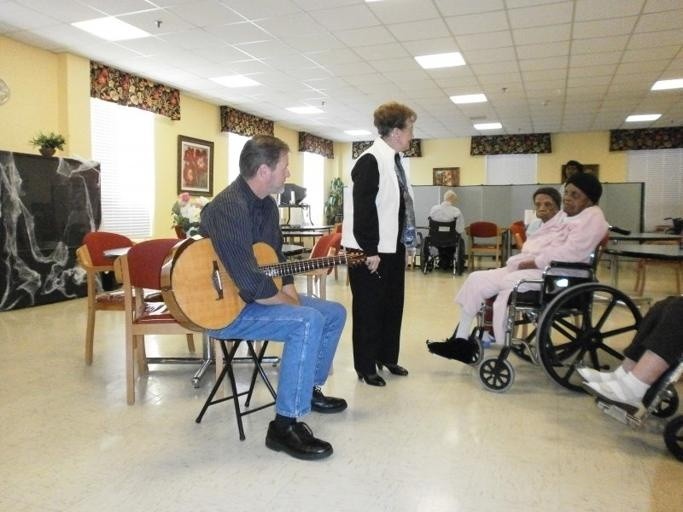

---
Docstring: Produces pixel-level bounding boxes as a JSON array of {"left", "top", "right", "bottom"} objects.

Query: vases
[{"left": 175, "top": 225, "right": 189, "bottom": 239}]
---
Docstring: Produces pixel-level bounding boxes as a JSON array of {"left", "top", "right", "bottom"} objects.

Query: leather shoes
[
  {"left": 263, "top": 422, "right": 334, "bottom": 460},
  {"left": 312, "top": 387, "right": 352, "bottom": 414}
]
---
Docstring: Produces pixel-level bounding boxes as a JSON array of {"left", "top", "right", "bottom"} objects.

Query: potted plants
[{"left": 28, "top": 131, "right": 65, "bottom": 157}]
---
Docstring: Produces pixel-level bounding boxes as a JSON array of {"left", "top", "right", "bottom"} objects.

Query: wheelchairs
[
  {"left": 594, "top": 359, "right": 683, "bottom": 464},
  {"left": 468, "top": 224, "right": 643, "bottom": 392},
  {"left": 419, "top": 217, "right": 465, "bottom": 275}
]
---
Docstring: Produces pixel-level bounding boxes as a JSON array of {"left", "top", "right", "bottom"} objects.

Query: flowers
[{"left": 170, "top": 192, "right": 209, "bottom": 225}]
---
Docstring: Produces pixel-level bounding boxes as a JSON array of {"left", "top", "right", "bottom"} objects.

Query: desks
[
  {"left": 103, "top": 244, "right": 305, "bottom": 389},
  {"left": 277, "top": 202, "right": 316, "bottom": 246},
  {"left": 594, "top": 231, "right": 683, "bottom": 308},
  {"left": 277, "top": 224, "right": 335, "bottom": 231},
  {"left": 280, "top": 231, "right": 324, "bottom": 245}
]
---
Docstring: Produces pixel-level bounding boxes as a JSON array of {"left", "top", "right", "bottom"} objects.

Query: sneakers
[{"left": 429, "top": 337, "right": 474, "bottom": 364}]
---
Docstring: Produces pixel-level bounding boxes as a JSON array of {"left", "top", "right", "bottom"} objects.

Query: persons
[
  {"left": 198, "top": 133, "right": 347, "bottom": 461},
  {"left": 474, "top": 187, "right": 562, "bottom": 348},
  {"left": 557, "top": 160, "right": 585, "bottom": 211},
  {"left": 427, "top": 173, "right": 609, "bottom": 365},
  {"left": 429, "top": 190, "right": 465, "bottom": 272},
  {"left": 340, "top": 103, "right": 418, "bottom": 387},
  {"left": 576, "top": 295, "right": 683, "bottom": 409}
]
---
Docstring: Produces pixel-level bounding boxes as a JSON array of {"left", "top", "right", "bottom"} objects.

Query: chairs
[
  {"left": 293, "top": 228, "right": 343, "bottom": 299},
  {"left": 113, "top": 239, "right": 230, "bottom": 404},
  {"left": 76, "top": 232, "right": 194, "bottom": 365},
  {"left": 467, "top": 222, "right": 500, "bottom": 269}
]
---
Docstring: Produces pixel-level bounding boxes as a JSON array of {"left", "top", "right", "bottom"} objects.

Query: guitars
[{"left": 159, "top": 237, "right": 369, "bottom": 330}]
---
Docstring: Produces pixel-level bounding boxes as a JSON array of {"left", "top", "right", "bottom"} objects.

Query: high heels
[
  {"left": 378, "top": 360, "right": 411, "bottom": 376},
  {"left": 355, "top": 371, "right": 386, "bottom": 387}
]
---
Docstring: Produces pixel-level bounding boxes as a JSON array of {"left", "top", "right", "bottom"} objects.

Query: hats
[
  {"left": 564, "top": 171, "right": 603, "bottom": 205},
  {"left": 533, "top": 186, "right": 561, "bottom": 209}
]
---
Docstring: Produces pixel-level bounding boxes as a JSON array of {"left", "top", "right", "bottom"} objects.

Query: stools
[{"left": 196, "top": 332, "right": 284, "bottom": 441}]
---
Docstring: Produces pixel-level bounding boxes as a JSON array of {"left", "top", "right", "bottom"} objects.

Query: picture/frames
[
  {"left": 433, "top": 167, "right": 460, "bottom": 186},
  {"left": 177, "top": 135, "right": 214, "bottom": 197}
]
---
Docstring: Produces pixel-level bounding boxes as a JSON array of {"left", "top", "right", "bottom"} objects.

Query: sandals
[
  {"left": 585, "top": 380, "right": 647, "bottom": 409},
  {"left": 577, "top": 367, "right": 617, "bottom": 383}
]
